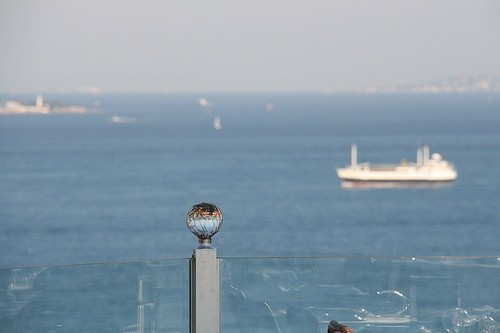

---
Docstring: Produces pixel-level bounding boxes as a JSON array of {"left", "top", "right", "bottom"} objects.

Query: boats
[{"left": 337, "top": 144, "right": 458, "bottom": 181}]
